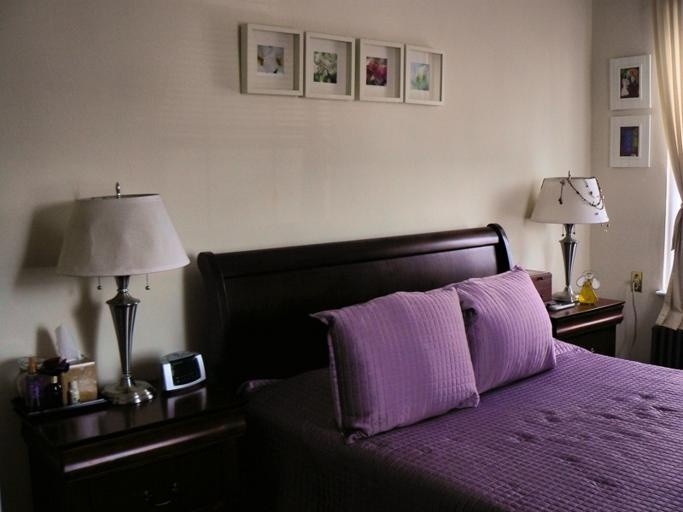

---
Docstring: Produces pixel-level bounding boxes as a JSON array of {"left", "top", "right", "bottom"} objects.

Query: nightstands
[
  {"left": 545, "top": 296, "right": 627, "bottom": 356},
  {"left": 20, "top": 382, "right": 249, "bottom": 511}
]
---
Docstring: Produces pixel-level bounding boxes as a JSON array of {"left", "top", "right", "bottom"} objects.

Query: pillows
[
  {"left": 441, "top": 265, "right": 558, "bottom": 394},
  {"left": 309, "top": 285, "right": 480, "bottom": 445}
]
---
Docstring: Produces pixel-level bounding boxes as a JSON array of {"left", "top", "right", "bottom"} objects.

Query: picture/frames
[
  {"left": 240, "top": 22, "right": 304, "bottom": 96},
  {"left": 303, "top": 31, "right": 356, "bottom": 102},
  {"left": 608, "top": 53, "right": 652, "bottom": 110},
  {"left": 355, "top": 38, "right": 406, "bottom": 103},
  {"left": 404, "top": 43, "right": 445, "bottom": 107},
  {"left": 609, "top": 113, "right": 653, "bottom": 168}
]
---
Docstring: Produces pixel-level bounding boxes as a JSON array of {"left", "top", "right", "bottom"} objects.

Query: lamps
[
  {"left": 55, "top": 182, "right": 191, "bottom": 407},
  {"left": 530, "top": 176, "right": 611, "bottom": 304}
]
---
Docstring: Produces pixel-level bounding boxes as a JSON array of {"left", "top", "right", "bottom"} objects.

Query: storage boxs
[{"left": 525, "top": 269, "right": 552, "bottom": 301}]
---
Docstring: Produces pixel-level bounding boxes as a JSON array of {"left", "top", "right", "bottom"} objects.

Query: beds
[{"left": 195, "top": 223, "right": 682, "bottom": 512}]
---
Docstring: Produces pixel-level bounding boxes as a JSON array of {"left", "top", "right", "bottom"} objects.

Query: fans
[{"left": 575, "top": 272, "right": 600, "bottom": 305}]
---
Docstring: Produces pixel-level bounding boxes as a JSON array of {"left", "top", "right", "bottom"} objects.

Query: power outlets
[{"left": 631, "top": 271, "right": 643, "bottom": 292}]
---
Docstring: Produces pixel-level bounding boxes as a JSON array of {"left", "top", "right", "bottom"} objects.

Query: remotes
[{"left": 549, "top": 302, "right": 575, "bottom": 310}]
